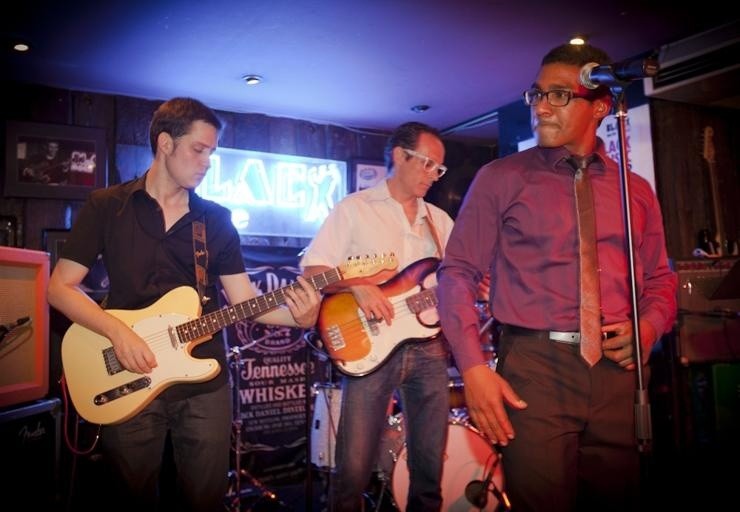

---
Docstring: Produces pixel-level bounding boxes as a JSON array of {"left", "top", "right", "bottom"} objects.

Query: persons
[
  {"left": 20, "top": 140, "right": 74, "bottom": 187},
  {"left": 45, "top": 95, "right": 326, "bottom": 511},
  {"left": 432, "top": 40, "right": 680, "bottom": 510},
  {"left": 285, "top": 119, "right": 495, "bottom": 511}
]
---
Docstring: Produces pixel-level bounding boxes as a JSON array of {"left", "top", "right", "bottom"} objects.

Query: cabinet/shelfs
[{"left": 670, "top": 257, "right": 739, "bottom": 454}]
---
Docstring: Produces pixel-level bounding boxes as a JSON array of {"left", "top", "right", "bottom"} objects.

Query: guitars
[
  {"left": 315, "top": 257, "right": 490, "bottom": 378},
  {"left": 692, "top": 127, "right": 738, "bottom": 258},
  {"left": 61, "top": 251, "right": 399, "bottom": 424}
]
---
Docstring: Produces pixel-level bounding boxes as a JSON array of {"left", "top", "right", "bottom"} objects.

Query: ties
[{"left": 564, "top": 152, "right": 605, "bottom": 369}]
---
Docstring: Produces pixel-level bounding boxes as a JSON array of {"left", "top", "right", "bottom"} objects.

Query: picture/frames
[{"left": 43, "top": 228, "right": 74, "bottom": 278}]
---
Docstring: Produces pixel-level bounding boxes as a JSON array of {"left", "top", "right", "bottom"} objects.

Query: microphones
[
  {"left": 579, "top": 57, "right": 660, "bottom": 90},
  {"left": 714, "top": 305, "right": 740, "bottom": 319},
  {"left": 0, "top": 317, "right": 29, "bottom": 342}
]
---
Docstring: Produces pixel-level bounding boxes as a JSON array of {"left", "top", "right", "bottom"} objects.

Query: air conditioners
[{"left": 642, "top": 21, "right": 739, "bottom": 105}]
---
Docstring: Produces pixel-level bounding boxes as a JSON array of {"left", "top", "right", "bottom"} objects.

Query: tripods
[{"left": 226, "top": 352, "right": 286, "bottom": 512}]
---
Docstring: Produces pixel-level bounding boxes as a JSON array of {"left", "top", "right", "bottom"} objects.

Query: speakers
[
  {"left": 0, "top": 246, "right": 51, "bottom": 409},
  {"left": 671, "top": 298, "right": 740, "bottom": 499}
]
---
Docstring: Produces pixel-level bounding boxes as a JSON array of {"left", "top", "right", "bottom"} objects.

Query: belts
[{"left": 490, "top": 315, "right": 618, "bottom": 346}]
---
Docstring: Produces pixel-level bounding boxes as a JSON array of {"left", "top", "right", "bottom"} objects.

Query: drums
[
  {"left": 310, "top": 383, "right": 393, "bottom": 473},
  {"left": 447, "top": 383, "right": 466, "bottom": 412},
  {"left": 391, "top": 417, "right": 504, "bottom": 511}
]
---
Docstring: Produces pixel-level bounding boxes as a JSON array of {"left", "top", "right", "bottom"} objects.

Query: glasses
[
  {"left": 401, "top": 147, "right": 449, "bottom": 179},
  {"left": 522, "top": 88, "right": 598, "bottom": 108}
]
